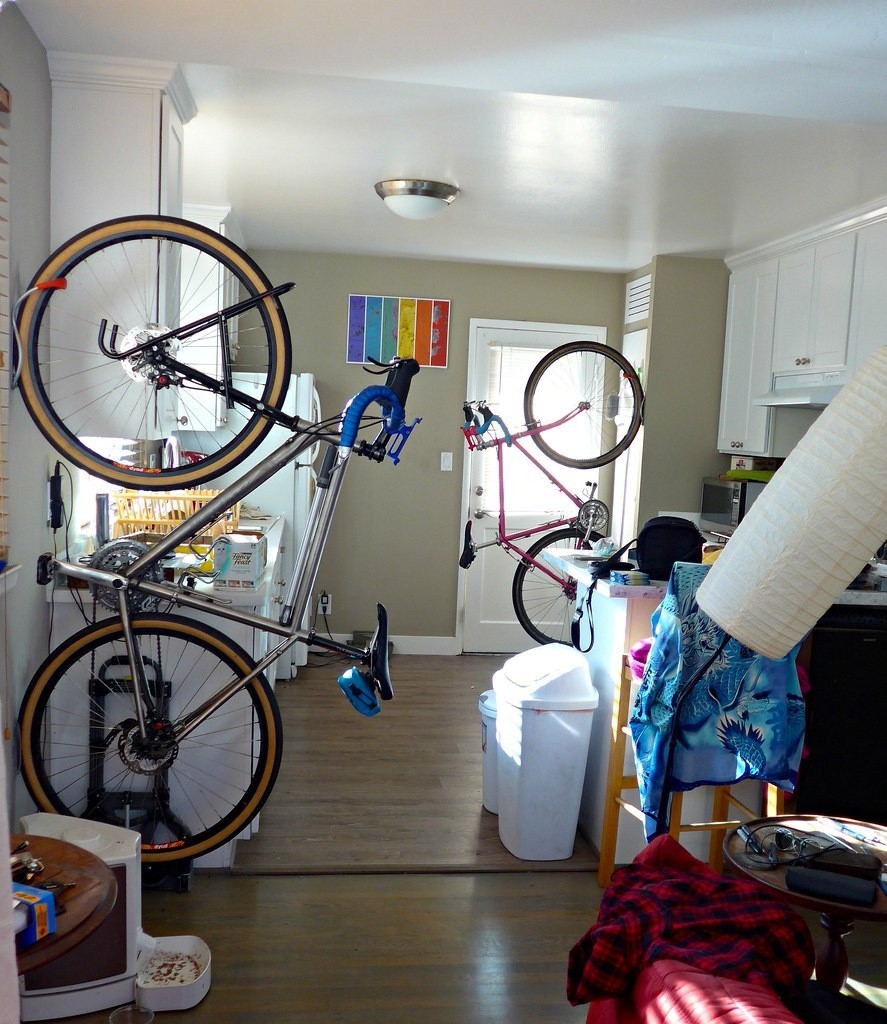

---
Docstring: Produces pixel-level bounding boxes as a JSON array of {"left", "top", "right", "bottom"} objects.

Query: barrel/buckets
[{"left": 479, "top": 690, "right": 499, "bottom": 816}]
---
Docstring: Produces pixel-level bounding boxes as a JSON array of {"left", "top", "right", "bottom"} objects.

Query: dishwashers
[{"left": 796, "top": 606, "right": 887, "bottom": 827}]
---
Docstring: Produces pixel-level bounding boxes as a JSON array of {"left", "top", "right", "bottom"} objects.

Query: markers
[{"left": 737, "top": 829, "right": 759, "bottom": 854}]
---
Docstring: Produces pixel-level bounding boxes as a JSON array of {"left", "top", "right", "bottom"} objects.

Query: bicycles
[
  {"left": 459, "top": 340, "right": 644, "bottom": 645},
  {"left": 4, "top": 209, "right": 424, "bottom": 869}
]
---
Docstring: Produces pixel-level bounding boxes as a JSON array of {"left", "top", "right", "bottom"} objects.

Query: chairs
[{"left": 597, "top": 562, "right": 794, "bottom": 892}]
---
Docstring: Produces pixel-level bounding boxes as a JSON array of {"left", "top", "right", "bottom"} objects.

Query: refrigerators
[{"left": 171, "top": 372, "right": 324, "bottom": 681}]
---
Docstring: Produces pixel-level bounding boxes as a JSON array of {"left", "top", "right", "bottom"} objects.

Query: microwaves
[{"left": 699, "top": 476, "right": 770, "bottom": 530}]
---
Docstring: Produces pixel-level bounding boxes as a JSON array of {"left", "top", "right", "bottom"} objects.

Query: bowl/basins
[{"left": 134, "top": 935, "right": 213, "bottom": 1011}]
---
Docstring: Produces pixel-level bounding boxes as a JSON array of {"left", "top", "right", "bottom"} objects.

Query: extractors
[{"left": 751, "top": 373, "right": 846, "bottom": 409}]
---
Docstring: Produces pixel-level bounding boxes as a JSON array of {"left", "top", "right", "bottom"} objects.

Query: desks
[
  {"left": 541, "top": 548, "right": 886, "bottom": 605},
  {"left": 44, "top": 515, "right": 286, "bottom": 870}
]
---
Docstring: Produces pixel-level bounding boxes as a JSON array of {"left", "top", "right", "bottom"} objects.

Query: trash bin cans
[
  {"left": 491, "top": 642, "right": 600, "bottom": 861},
  {"left": 476, "top": 687, "right": 497, "bottom": 812}
]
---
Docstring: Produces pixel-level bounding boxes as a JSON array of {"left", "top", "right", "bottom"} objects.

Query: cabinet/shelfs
[
  {"left": 716, "top": 253, "right": 826, "bottom": 459},
  {"left": 770, "top": 229, "right": 858, "bottom": 387}
]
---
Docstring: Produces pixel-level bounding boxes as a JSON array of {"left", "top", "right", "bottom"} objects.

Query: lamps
[{"left": 373, "top": 178, "right": 463, "bottom": 221}]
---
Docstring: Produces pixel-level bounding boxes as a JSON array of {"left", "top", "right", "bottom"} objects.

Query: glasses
[{"left": 773, "top": 827, "right": 808, "bottom": 856}]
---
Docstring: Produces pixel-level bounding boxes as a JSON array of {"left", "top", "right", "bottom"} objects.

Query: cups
[{"left": 109, "top": 1005, "right": 154, "bottom": 1024}]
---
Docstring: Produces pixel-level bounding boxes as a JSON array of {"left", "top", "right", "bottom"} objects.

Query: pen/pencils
[
  {"left": 817, "top": 817, "right": 877, "bottom": 847},
  {"left": 741, "top": 824, "right": 766, "bottom": 853}
]
